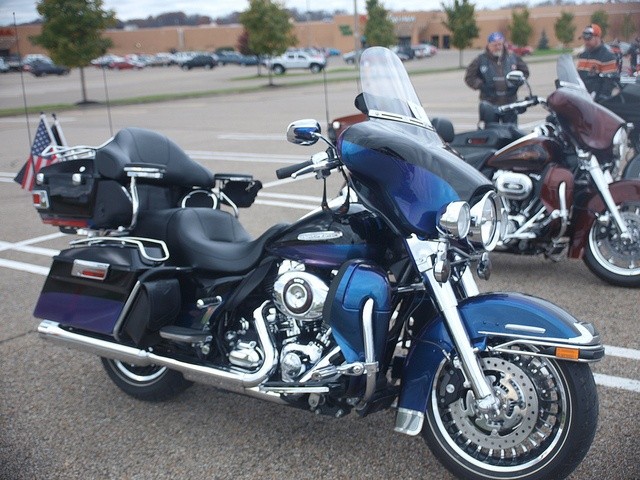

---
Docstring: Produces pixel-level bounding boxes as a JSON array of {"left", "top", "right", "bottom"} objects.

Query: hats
[
  {"left": 579, "top": 23, "right": 603, "bottom": 41},
  {"left": 487, "top": 31, "right": 506, "bottom": 43}
]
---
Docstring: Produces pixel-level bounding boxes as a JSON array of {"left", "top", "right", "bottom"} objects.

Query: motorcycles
[
  {"left": 33, "top": 46, "right": 605, "bottom": 480},
  {"left": 328, "top": 53, "right": 640, "bottom": 287}
]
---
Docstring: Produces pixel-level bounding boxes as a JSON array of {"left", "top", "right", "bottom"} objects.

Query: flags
[
  {"left": 21, "top": 118, "right": 57, "bottom": 193},
  {"left": 13, "top": 123, "right": 62, "bottom": 187}
]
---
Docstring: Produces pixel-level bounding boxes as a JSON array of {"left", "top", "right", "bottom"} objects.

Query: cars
[
  {"left": 21, "top": 54, "right": 55, "bottom": 72},
  {"left": 181, "top": 55, "right": 218, "bottom": 70},
  {"left": 343, "top": 48, "right": 362, "bottom": 64},
  {"left": 31, "top": 59, "right": 66, "bottom": 75},
  {"left": 217, "top": 51, "right": 258, "bottom": 66},
  {"left": 90, "top": 52, "right": 198, "bottom": 69},
  {"left": 505, "top": 41, "right": 532, "bottom": 56}
]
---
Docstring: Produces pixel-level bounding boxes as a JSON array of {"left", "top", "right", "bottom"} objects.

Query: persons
[
  {"left": 464, "top": 32, "right": 529, "bottom": 129},
  {"left": 609, "top": 38, "right": 623, "bottom": 72},
  {"left": 575, "top": 24, "right": 621, "bottom": 108},
  {"left": 628, "top": 37, "right": 640, "bottom": 73}
]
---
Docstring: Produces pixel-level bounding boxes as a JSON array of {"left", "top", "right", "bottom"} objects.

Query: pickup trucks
[{"left": 262, "top": 51, "right": 327, "bottom": 74}]
